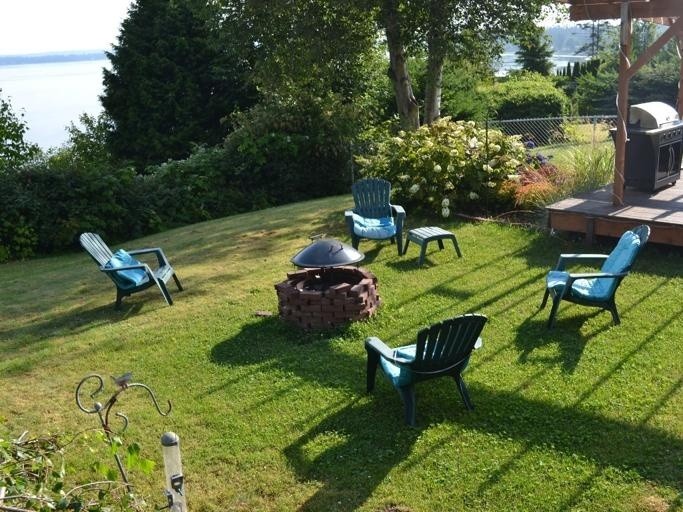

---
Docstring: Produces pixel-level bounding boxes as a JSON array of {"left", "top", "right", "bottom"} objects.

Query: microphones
[{"left": 403, "top": 225, "right": 462, "bottom": 267}]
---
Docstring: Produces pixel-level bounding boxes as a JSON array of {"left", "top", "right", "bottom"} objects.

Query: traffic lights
[{"left": 106, "top": 248, "right": 148, "bottom": 287}]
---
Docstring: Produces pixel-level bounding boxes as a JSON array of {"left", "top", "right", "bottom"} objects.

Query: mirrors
[{"left": 108, "top": 371, "right": 134, "bottom": 390}]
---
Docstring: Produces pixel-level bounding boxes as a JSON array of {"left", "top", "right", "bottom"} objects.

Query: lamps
[
  {"left": 364, "top": 312, "right": 487, "bottom": 427},
  {"left": 79, "top": 232, "right": 182, "bottom": 310},
  {"left": 344, "top": 177, "right": 405, "bottom": 256},
  {"left": 541, "top": 221, "right": 651, "bottom": 331}
]
